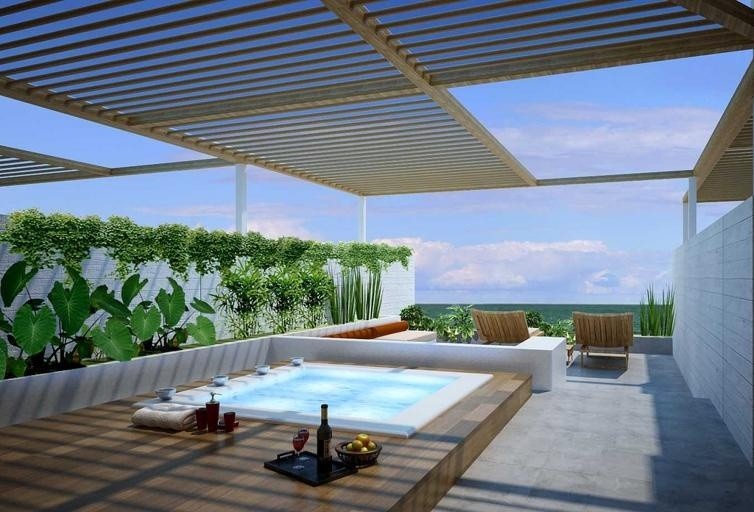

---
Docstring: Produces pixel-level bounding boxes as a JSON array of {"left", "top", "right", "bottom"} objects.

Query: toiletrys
[{"left": 206, "top": 392, "right": 221, "bottom": 431}]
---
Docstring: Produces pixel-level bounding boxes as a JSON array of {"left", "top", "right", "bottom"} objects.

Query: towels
[{"left": 131, "top": 403, "right": 199, "bottom": 431}]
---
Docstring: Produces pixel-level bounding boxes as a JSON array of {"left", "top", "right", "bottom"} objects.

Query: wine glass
[{"left": 291, "top": 428, "right": 310, "bottom": 471}]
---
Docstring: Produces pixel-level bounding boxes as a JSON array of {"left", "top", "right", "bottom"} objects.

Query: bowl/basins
[
  {"left": 335, "top": 440, "right": 383, "bottom": 469},
  {"left": 254, "top": 365, "right": 272, "bottom": 375},
  {"left": 154, "top": 387, "right": 177, "bottom": 401},
  {"left": 291, "top": 356, "right": 305, "bottom": 366},
  {"left": 213, "top": 375, "right": 230, "bottom": 387}
]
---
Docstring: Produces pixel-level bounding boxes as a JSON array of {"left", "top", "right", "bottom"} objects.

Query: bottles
[{"left": 316, "top": 404, "right": 333, "bottom": 474}]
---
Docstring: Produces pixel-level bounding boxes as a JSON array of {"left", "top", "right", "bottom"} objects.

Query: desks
[{"left": 566, "top": 344, "right": 574, "bottom": 365}]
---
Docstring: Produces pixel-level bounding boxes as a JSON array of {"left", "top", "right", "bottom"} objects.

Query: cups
[
  {"left": 195, "top": 408, "right": 208, "bottom": 431},
  {"left": 223, "top": 411, "right": 237, "bottom": 433}
]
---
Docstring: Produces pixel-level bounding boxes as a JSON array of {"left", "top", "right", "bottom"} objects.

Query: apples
[{"left": 347, "top": 434, "right": 376, "bottom": 452}]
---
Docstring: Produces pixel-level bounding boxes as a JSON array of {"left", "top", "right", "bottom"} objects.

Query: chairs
[
  {"left": 572, "top": 311, "right": 633, "bottom": 369},
  {"left": 470, "top": 308, "right": 544, "bottom": 346}
]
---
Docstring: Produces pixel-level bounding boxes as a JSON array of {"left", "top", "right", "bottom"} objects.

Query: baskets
[{"left": 334, "top": 440, "right": 382, "bottom": 465}]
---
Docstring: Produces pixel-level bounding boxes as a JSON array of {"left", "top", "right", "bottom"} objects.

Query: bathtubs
[{"left": 130, "top": 361, "right": 494, "bottom": 442}]
